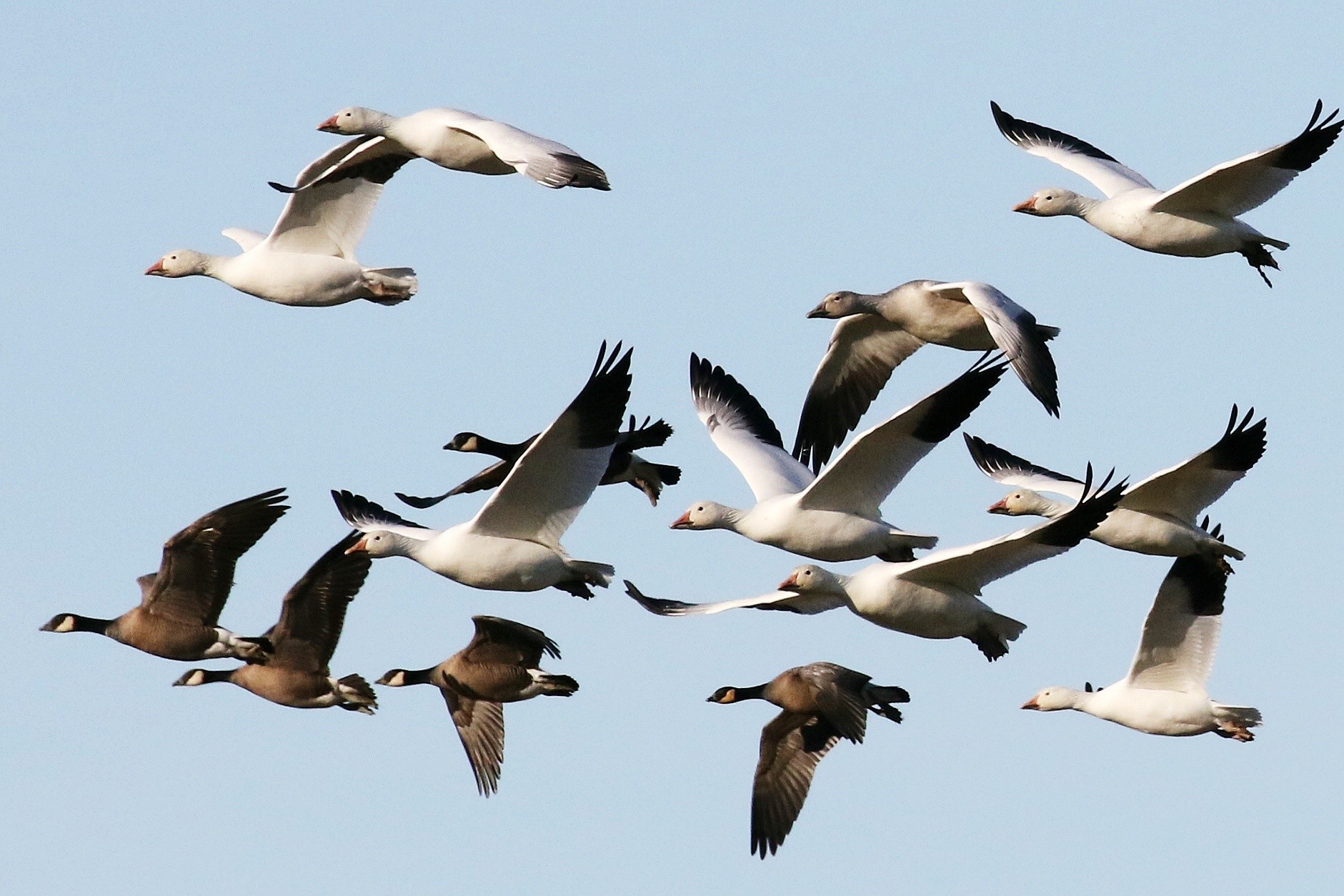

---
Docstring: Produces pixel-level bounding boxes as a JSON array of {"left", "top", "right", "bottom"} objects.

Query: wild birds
[
  {"left": 394, "top": 416, "right": 682, "bottom": 509},
  {"left": 144, "top": 134, "right": 421, "bottom": 306},
  {"left": 170, "top": 527, "right": 381, "bottom": 719},
  {"left": 624, "top": 280, "right": 1268, "bottom": 665},
  {"left": 705, "top": 660, "right": 911, "bottom": 862},
  {"left": 266, "top": 106, "right": 614, "bottom": 195},
  {"left": 332, "top": 340, "right": 637, "bottom": 600},
  {"left": 38, "top": 486, "right": 293, "bottom": 662},
  {"left": 989, "top": 99, "right": 1343, "bottom": 290},
  {"left": 1021, "top": 514, "right": 1263, "bottom": 743},
  {"left": 375, "top": 614, "right": 581, "bottom": 799}
]
